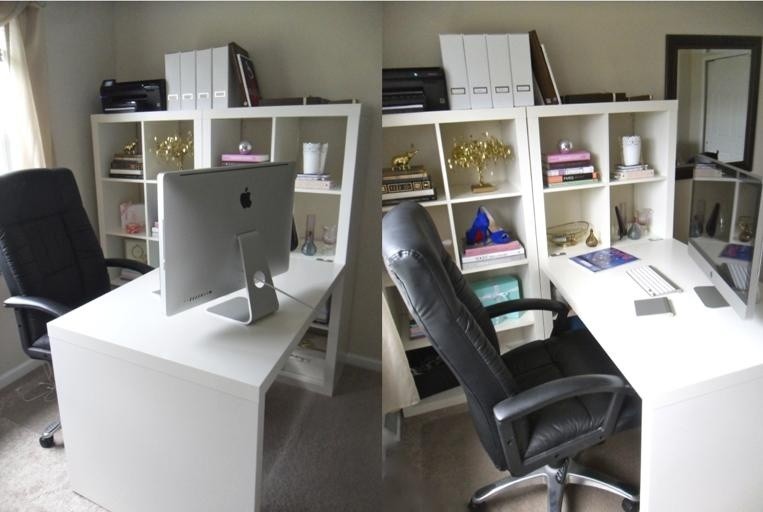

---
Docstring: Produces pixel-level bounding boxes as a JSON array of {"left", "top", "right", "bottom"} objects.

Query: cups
[
  {"left": 618, "top": 135, "right": 642, "bottom": 166},
  {"left": 303, "top": 142, "right": 329, "bottom": 175}
]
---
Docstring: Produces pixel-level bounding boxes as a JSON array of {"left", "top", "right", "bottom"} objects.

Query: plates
[{"left": 547, "top": 220, "right": 588, "bottom": 248}]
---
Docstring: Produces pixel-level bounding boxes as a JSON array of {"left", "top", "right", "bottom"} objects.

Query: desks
[
  {"left": 539, "top": 230, "right": 763, "bottom": 512},
  {"left": 46, "top": 232, "right": 347, "bottom": 512}
]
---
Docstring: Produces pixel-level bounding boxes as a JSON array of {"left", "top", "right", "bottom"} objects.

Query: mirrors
[{"left": 665, "top": 33, "right": 762, "bottom": 181}]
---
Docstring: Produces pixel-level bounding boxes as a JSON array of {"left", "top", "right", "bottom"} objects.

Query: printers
[
  {"left": 381, "top": 67, "right": 449, "bottom": 114},
  {"left": 99, "top": 78, "right": 165, "bottom": 114}
]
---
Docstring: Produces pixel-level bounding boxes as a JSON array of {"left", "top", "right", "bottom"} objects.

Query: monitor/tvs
[
  {"left": 688, "top": 154, "right": 762, "bottom": 319},
  {"left": 157, "top": 160, "right": 296, "bottom": 326}
]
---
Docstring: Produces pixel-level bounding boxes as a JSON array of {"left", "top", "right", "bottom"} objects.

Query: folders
[
  {"left": 463, "top": 34, "right": 493, "bottom": 110},
  {"left": 163, "top": 52, "right": 180, "bottom": 111},
  {"left": 180, "top": 50, "right": 196, "bottom": 112},
  {"left": 196, "top": 47, "right": 212, "bottom": 111},
  {"left": 486, "top": 34, "right": 514, "bottom": 108},
  {"left": 439, "top": 32, "right": 472, "bottom": 111},
  {"left": 212, "top": 45, "right": 240, "bottom": 109},
  {"left": 509, "top": 34, "right": 534, "bottom": 107}
]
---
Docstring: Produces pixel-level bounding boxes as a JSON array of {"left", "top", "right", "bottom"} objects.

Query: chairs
[
  {"left": 382, "top": 200, "right": 642, "bottom": 512},
  {"left": 0, "top": 166, "right": 155, "bottom": 449}
]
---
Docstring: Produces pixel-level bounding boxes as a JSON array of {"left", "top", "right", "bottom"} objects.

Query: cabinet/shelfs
[
  {"left": 89, "top": 104, "right": 375, "bottom": 399},
  {"left": 526, "top": 97, "right": 679, "bottom": 340},
  {"left": 382, "top": 106, "right": 546, "bottom": 420}
]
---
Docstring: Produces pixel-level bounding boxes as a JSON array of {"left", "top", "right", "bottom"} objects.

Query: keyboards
[{"left": 626, "top": 265, "right": 682, "bottom": 297}]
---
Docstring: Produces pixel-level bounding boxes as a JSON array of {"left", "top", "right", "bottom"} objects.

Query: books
[
  {"left": 293, "top": 173, "right": 337, "bottom": 190},
  {"left": 613, "top": 162, "right": 655, "bottom": 181},
  {"left": 461, "top": 240, "right": 526, "bottom": 269},
  {"left": 108, "top": 152, "right": 143, "bottom": 180},
  {"left": 543, "top": 150, "right": 600, "bottom": 188},
  {"left": 220, "top": 152, "right": 270, "bottom": 167},
  {"left": 376, "top": 165, "right": 439, "bottom": 206}
]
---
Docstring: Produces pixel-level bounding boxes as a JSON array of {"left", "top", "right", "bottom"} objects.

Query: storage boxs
[{"left": 469, "top": 275, "right": 521, "bottom": 326}]
